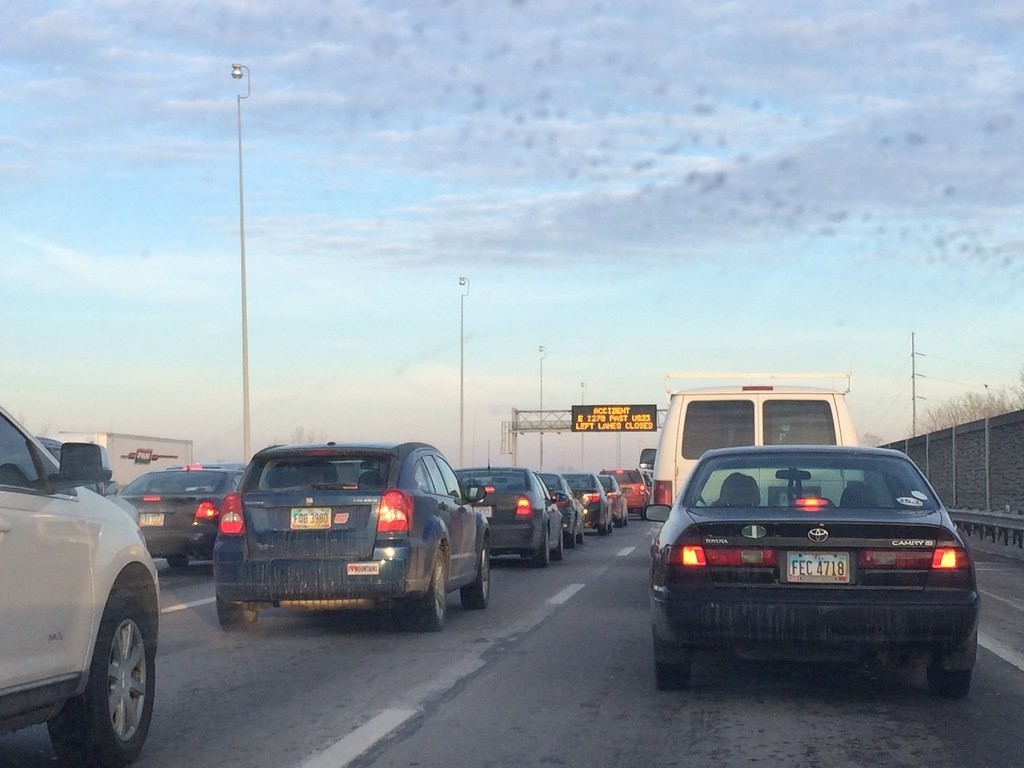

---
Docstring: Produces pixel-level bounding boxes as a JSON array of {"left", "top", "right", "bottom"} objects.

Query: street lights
[
  {"left": 580, "top": 382, "right": 587, "bottom": 473},
  {"left": 539, "top": 344, "right": 546, "bottom": 473},
  {"left": 459, "top": 276, "right": 470, "bottom": 470},
  {"left": 231, "top": 62, "right": 252, "bottom": 467}
]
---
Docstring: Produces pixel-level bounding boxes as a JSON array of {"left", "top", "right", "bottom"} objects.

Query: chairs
[
  {"left": 357, "top": 471, "right": 376, "bottom": 490},
  {"left": 725, "top": 476, "right": 761, "bottom": 506},
  {"left": 321, "top": 463, "right": 340, "bottom": 484},
  {"left": 839, "top": 484, "right": 878, "bottom": 508},
  {"left": 269, "top": 468, "right": 306, "bottom": 488}
]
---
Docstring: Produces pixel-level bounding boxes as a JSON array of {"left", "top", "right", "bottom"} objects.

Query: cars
[
  {"left": 561, "top": 472, "right": 614, "bottom": 537},
  {"left": 534, "top": 469, "right": 589, "bottom": 550},
  {"left": 454, "top": 467, "right": 571, "bottom": 570},
  {"left": 599, "top": 467, "right": 655, "bottom": 521},
  {"left": 641, "top": 443, "right": 982, "bottom": 697},
  {"left": 116, "top": 461, "right": 252, "bottom": 569},
  {"left": 0, "top": 401, "right": 163, "bottom": 768},
  {"left": 595, "top": 474, "right": 629, "bottom": 528},
  {"left": 209, "top": 440, "right": 495, "bottom": 635}
]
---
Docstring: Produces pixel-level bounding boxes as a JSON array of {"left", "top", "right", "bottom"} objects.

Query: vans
[{"left": 639, "top": 383, "right": 866, "bottom": 550}]
